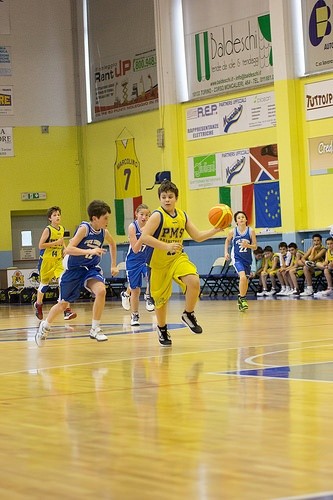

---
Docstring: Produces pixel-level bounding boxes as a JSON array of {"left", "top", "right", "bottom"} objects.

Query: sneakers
[
  {"left": 143, "top": 293, "right": 155, "bottom": 311},
  {"left": 130, "top": 311, "right": 140, "bottom": 325},
  {"left": 33, "top": 301, "right": 44, "bottom": 320},
  {"left": 181, "top": 310, "right": 202, "bottom": 334},
  {"left": 156, "top": 324, "right": 172, "bottom": 347},
  {"left": 64, "top": 308, "right": 77, "bottom": 320},
  {"left": 120, "top": 290, "right": 131, "bottom": 310}
]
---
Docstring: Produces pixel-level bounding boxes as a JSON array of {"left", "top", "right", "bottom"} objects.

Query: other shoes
[
  {"left": 264, "top": 288, "right": 277, "bottom": 295},
  {"left": 287, "top": 288, "right": 300, "bottom": 295},
  {"left": 277, "top": 285, "right": 290, "bottom": 295},
  {"left": 35, "top": 320, "right": 50, "bottom": 347},
  {"left": 256, "top": 289, "right": 268, "bottom": 297},
  {"left": 299, "top": 286, "right": 314, "bottom": 296},
  {"left": 236, "top": 293, "right": 248, "bottom": 311},
  {"left": 89, "top": 326, "right": 108, "bottom": 341}
]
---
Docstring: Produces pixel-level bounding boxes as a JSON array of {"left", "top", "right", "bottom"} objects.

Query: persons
[
  {"left": 225, "top": 211, "right": 258, "bottom": 313},
  {"left": 141, "top": 180, "right": 228, "bottom": 347},
  {"left": 121, "top": 204, "right": 155, "bottom": 326},
  {"left": 35, "top": 199, "right": 120, "bottom": 347},
  {"left": 249, "top": 233, "right": 333, "bottom": 297},
  {"left": 33, "top": 206, "right": 77, "bottom": 320}
]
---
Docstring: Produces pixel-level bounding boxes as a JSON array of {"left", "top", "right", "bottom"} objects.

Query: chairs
[
  {"left": 106, "top": 262, "right": 127, "bottom": 299},
  {"left": 199, "top": 257, "right": 333, "bottom": 297}
]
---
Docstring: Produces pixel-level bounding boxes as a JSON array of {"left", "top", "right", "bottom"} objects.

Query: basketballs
[{"left": 208, "top": 204, "right": 233, "bottom": 228}]
